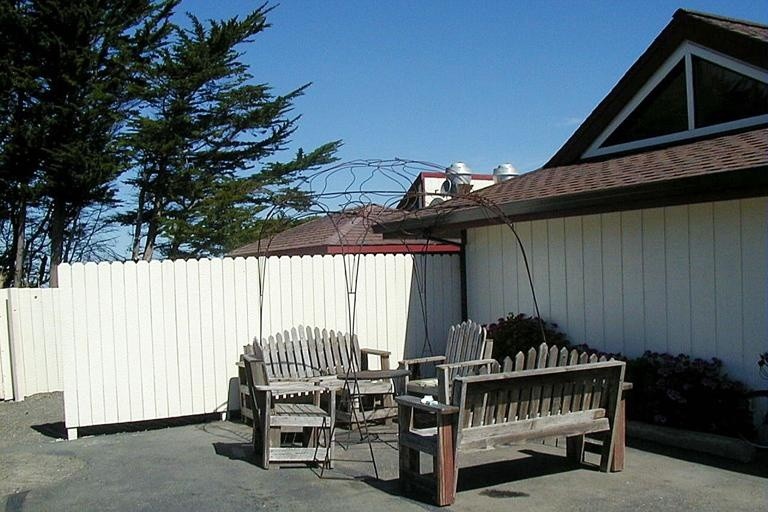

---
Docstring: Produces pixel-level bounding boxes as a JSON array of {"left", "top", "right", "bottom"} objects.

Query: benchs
[
  {"left": 236, "top": 325, "right": 396, "bottom": 432},
  {"left": 394, "top": 342, "right": 634, "bottom": 507}
]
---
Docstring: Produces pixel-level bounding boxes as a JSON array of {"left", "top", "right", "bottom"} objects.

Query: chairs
[
  {"left": 243, "top": 336, "right": 336, "bottom": 468},
  {"left": 398, "top": 317, "right": 494, "bottom": 405}
]
